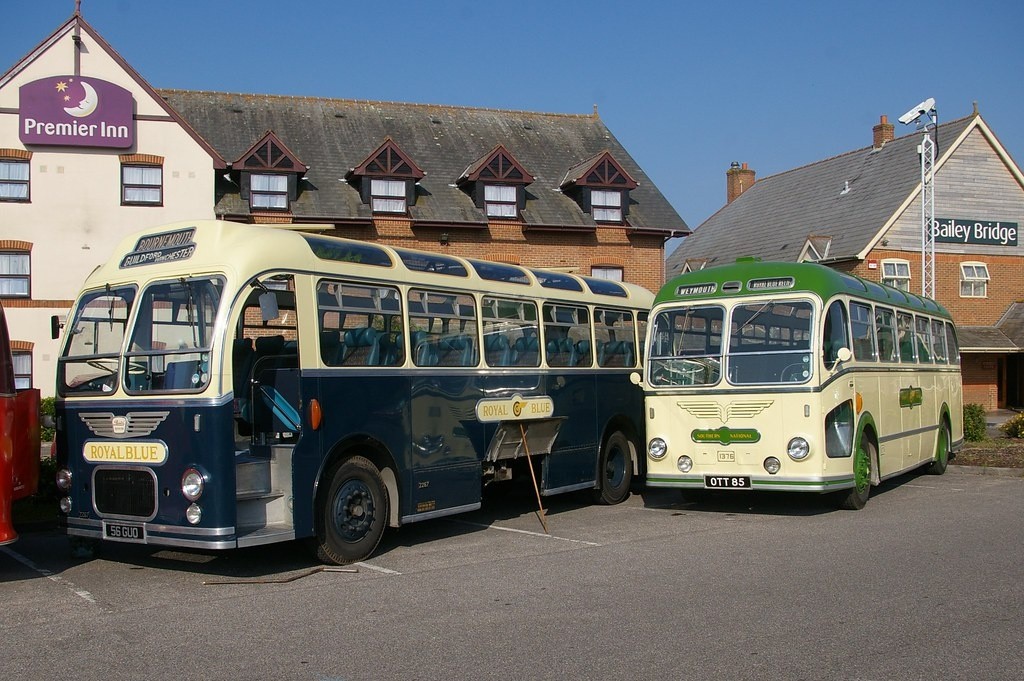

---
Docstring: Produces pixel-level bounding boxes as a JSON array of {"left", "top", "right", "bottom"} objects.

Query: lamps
[
  {"left": 72, "top": 35, "right": 81, "bottom": 41},
  {"left": 438, "top": 234, "right": 448, "bottom": 242}
]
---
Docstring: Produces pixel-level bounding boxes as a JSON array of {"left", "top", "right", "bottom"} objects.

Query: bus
[
  {"left": 629, "top": 254, "right": 965, "bottom": 510},
  {"left": 42, "top": 219, "right": 674, "bottom": 566}
]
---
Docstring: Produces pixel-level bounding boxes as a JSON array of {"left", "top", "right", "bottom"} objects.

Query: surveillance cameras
[
  {"left": 898, "top": 103, "right": 925, "bottom": 125},
  {"left": 918, "top": 98, "right": 935, "bottom": 114}
]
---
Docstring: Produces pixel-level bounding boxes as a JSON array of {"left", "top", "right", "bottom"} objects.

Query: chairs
[
  {"left": 232, "top": 328, "right": 632, "bottom": 368},
  {"left": 796, "top": 338, "right": 944, "bottom": 363}
]
